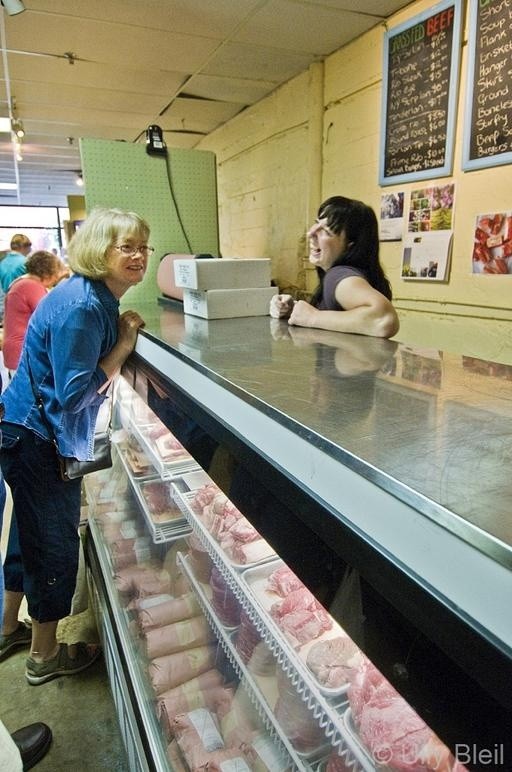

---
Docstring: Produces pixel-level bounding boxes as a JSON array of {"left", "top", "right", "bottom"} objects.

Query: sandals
[{"left": 0, "top": 619, "right": 101, "bottom": 686}]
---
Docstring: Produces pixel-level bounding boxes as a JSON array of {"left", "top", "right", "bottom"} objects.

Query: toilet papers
[{"left": 156, "top": 253, "right": 195, "bottom": 303}]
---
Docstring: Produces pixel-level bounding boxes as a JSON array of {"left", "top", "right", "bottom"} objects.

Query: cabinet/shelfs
[{"left": 74, "top": 328, "right": 512, "bottom": 771}]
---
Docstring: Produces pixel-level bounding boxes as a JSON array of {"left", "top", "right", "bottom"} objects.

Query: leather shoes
[{"left": 10, "top": 722, "right": 52, "bottom": 771}]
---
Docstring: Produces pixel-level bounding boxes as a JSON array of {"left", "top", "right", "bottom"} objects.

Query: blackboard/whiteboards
[{"left": 380, "top": 1, "right": 511, "bottom": 188}]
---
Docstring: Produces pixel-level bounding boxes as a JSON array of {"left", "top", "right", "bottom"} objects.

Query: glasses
[{"left": 112, "top": 245, "right": 155, "bottom": 255}]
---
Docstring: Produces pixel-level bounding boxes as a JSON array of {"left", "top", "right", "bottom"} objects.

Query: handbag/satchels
[{"left": 60, "top": 431, "right": 112, "bottom": 482}]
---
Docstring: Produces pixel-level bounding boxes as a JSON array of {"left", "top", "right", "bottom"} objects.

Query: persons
[{"left": 269, "top": 196, "right": 400, "bottom": 338}]
